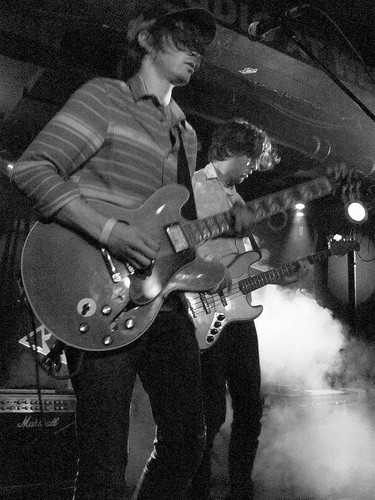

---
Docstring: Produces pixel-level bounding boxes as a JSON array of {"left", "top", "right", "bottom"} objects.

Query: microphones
[{"left": 248, "top": 3, "right": 311, "bottom": 41}]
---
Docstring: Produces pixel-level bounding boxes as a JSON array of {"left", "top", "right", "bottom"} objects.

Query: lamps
[{"left": 345, "top": 197, "right": 375, "bottom": 224}]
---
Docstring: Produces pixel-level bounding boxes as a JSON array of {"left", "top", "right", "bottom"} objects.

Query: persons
[
  {"left": 9, "top": 1, "right": 258, "bottom": 500},
  {"left": 182, "top": 116, "right": 315, "bottom": 500}
]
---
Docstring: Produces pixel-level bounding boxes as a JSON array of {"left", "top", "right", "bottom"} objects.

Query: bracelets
[{"left": 99, "top": 217, "right": 117, "bottom": 245}]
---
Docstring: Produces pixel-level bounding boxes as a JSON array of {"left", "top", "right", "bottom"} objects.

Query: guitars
[
  {"left": 185, "top": 237, "right": 361, "bottom": 351},
  {"left": 21, "top": 163, "right": 362, "bottom": 351}
]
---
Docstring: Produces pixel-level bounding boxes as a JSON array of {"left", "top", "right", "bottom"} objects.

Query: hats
[{"left": 126, "top": 1, "right": 217, "bottom": 44}]
